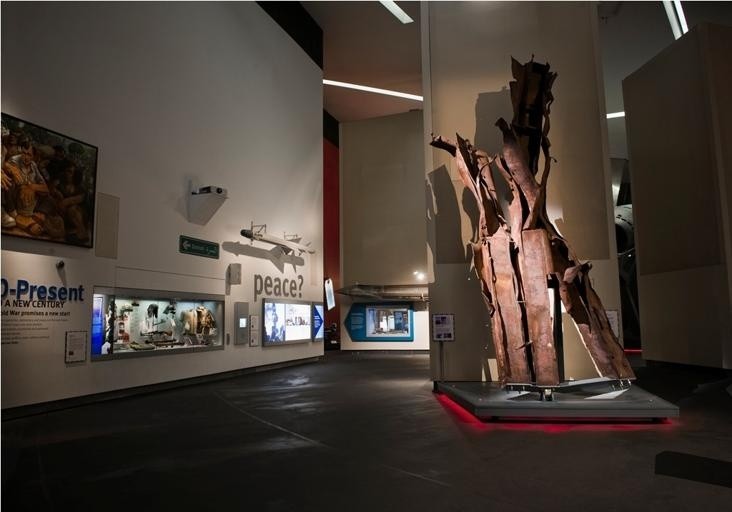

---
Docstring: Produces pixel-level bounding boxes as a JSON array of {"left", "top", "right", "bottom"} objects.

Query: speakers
[{"left": 230, "top": 263, "right": 241, "bottom": 284}]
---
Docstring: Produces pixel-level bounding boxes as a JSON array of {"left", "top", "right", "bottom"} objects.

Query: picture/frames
[
  {"left": 431, "top": 313, "right": 455, "bottom": 341},
  {"left": 1, "top": 111, "right": 98, "bottom": 249},
  {"left": 343, "top": 301, "right": 414, "bottom": 342}
]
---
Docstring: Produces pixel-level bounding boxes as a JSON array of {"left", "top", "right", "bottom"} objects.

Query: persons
[{"left": 1, "top": 124, "right": 87, "bottom": 242}]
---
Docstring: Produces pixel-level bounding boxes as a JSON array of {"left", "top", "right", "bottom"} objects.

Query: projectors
[{"left": 199, "top": 186, "right": 227, "bottom": 196}]
